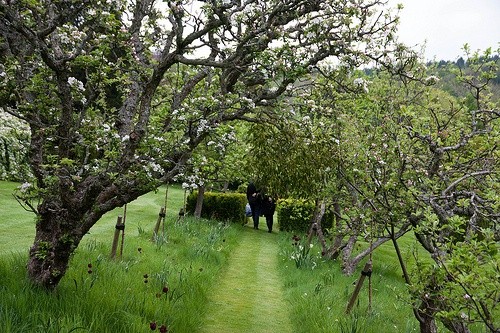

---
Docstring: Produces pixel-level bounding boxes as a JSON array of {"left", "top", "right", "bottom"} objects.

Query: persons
[
  {"left": 264, "top": 196, "right": 275, "bottom": 232},
  {"left": 250, "top": 192, "right": 261, "bottom": 230}
]
